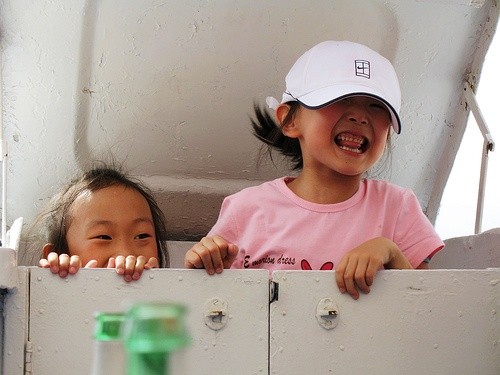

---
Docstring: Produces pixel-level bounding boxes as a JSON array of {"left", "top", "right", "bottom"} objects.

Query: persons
[
  {"left": 37, "top": 166, "right": 170, "bottom": 283},
  {"left": 183, "top": 39, "right": 446, "bottom": 300}
]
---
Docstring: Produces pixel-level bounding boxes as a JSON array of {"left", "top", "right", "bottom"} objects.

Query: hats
[{"left": 281, "top": 40, "right": 402, "bottom": 135}]
